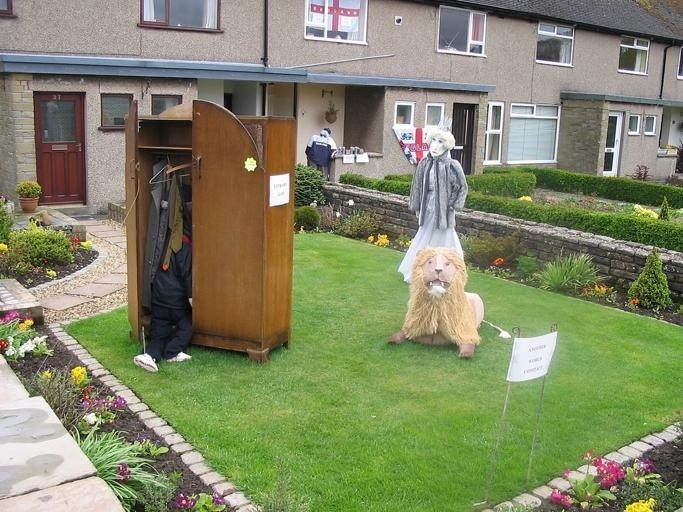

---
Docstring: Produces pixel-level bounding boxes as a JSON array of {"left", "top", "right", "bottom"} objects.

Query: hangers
[{"left": 148, "top": 150, "right": 191, "bottom": 185}]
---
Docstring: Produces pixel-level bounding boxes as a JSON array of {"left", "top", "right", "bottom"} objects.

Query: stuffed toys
[{"left": 389, "top": 245, "right": 511, "bottom": 358}]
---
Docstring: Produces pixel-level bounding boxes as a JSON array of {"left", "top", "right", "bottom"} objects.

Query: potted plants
[
  {"left": 14, "top": 179, "right": 43, "bottom": 213},
  {"left": 323, "top": 101, "right": 338, "bottom": 125}
]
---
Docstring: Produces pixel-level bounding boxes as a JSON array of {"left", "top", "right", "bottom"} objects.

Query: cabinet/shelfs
[{"left": 123, "top": 98, "right": 298, "bottom": 364}]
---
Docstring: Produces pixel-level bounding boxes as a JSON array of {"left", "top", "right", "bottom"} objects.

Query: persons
[
  {"left": 398, "top": 115, "right": 468, "bottom": 285},
  {"left": 305, "top": 127, "right": 337, "bottom": 180},
  {"left": 134, "top": 224, "right": 192, "bottom": 371}
]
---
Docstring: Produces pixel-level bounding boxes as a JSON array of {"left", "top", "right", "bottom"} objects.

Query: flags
[{"left": 307, "top": 0, "right": 361, "bottom": 33}]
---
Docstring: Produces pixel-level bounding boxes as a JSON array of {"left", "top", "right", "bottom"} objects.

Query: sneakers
[
  {"left": 133, "top": 353, "right": 159, "bottom": 373},
  {"left": 166, "top": 350, "right": 193, "bottom": 362}
]
